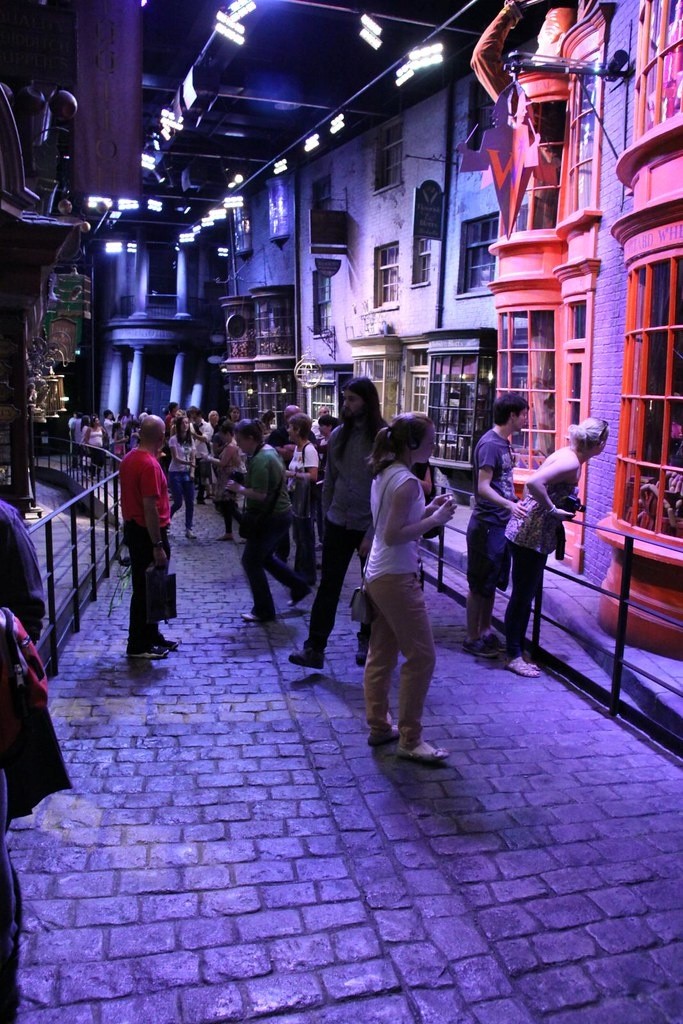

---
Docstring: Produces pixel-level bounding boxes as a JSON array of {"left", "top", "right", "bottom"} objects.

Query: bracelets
[{"left": 547, "top": 505, "right": 556, "bottom": 513}]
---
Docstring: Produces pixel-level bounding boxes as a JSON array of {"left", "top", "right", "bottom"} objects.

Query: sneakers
[
  {"left": 126, "top": 639, "right": 179, "bottom": 659},
  {"left": 462, "top": 633, "right": 506, "bottom": 657}
]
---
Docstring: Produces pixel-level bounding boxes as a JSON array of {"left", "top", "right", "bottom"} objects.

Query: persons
[
  {"left": 120, "top": 415, "right": 179, "bottom": 658},
  {"left": 506, "top": 417, "right": 609, "bottom": 678},
  {"left": 364, "top": 412, "right": 457, "bottom": 758},
  {"left": 68, "top": 401, "right": 341, "bottom": 587},
  {"left": 0, "top": 503, "right": 46, "bottom": 1024},
  {"left": 226, "top": 418, "right": 311, "bottom": 620},
  {"left": 466, "top": 394, "right": 527, "bottom": 657},
  {"left": 288, "top": 377, "right": 389, "bottom": 668}
]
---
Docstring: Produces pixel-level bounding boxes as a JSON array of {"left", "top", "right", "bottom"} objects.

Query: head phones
[{"left": 407, "top": 417, "right": 419, "bottom": 450}]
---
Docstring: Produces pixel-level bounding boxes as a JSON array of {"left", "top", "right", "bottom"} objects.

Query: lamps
[
  {"left": 142, "top": 151, "right": 156, "bottom": 169},
  {"left": 304, "top": 129, "right": 328, "bottom": 158},
  {"left": 329, "top": 112, "right": 351, "bottom": 138},
  {"left": 355, "top": 8, "right": 446, "bottom": 90},
  {"left": 211, "top": 0, "right": 257, "bottom": 49},
  {"left": 181, "top": 61, "right": 221, "bottom": 114}
]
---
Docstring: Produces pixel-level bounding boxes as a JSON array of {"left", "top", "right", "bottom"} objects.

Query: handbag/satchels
[
  {"left": 145, "top": 561, "right": 176, "bottom": 623},
  {"left": 292, "top": 473, "right": 316, "bottom": 521},
  {"left": 350, "top": 586, "right": 369, "bottom": 624},
  {"left": 3, "top": 607, "right": 73, "bottom": 820},
  {"left": 240, "top": 507, "right": 272, "bottom": 542}
]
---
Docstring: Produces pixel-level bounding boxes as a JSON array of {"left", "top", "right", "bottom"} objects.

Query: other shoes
[
  {"left": 287, "top": 641, "right": 325, "bottom": 669},
  {"left": 503, "top": 657, "right": 541, "bottom": 678},
  {"left": 355, "top": 644, "right": 368, "bottom": 666},
  {"left": 367, "top": 730, "right": 401, "bottom": 746},
  {"left": 215, "top": 534, "right": 236, "bottom": 542},
  {"left": 395, "top": 742, "right": 449, "bottom": 760},
  {"left": 184, "top": 532, "right": 197, "bottom": 539},
  {"left": 287, "top": 584, "right": 312, "bottom": 605},
  {"left": 242, "top": 612, "right": 276, "bottom": 621},
  {"left": 314, "top": 543, "right": 323, "bottom": 551}
]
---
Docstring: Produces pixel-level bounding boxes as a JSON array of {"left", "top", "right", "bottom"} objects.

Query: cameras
[
  {"left": 565, "top": 495, "right": 586, "bottom": 520},
  {"left": 225, "top": 470, "right": 245, "bottom": 497}
]
